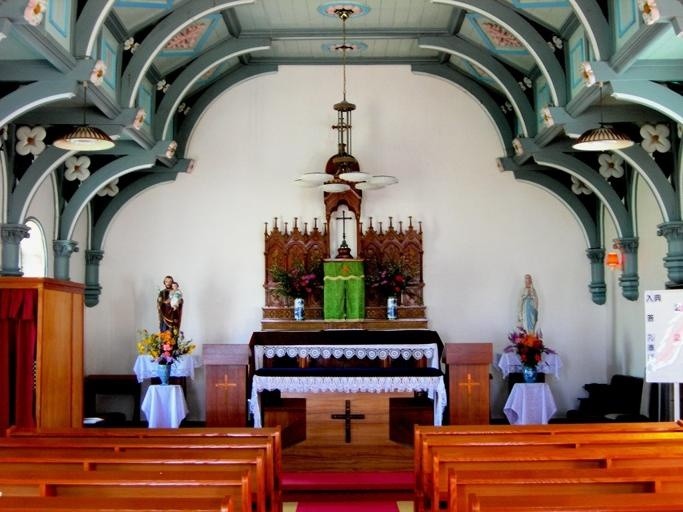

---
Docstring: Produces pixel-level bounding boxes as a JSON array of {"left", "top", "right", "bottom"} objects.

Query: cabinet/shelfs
[{"left": 0, "top": 276, "right": 89, "bottom": 429}]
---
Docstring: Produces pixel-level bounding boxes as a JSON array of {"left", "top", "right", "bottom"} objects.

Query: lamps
[
  {"left": 571, "top": 79, "right": 635, "bottom": 151},
  {"left": 50, "top": 79, "right": 117, "bottom": 153},
  {"left": 604, "top": 251, "right": 623, "bottom": 272},
  {"left": 296, "top": 0, "right": 400, "bottom": 196}
]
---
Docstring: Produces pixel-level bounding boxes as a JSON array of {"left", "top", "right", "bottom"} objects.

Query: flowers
[
  {"left": 134, "top": 328, "right": 197, "bottom": 366},
  {"left": 266, "top": 261, "right": 322, "bottom": 299},
  {"left": 502, "top": 327, "right": 557, "bottom": 372},
  {"left": 365, "top": 261, "right": 419, "bottom": 300}
]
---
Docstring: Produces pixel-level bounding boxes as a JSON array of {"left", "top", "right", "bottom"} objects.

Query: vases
[
  {"left": 522, "top": 367, "right": 537, "bottom": 382},
  {"left": 294, "top": 299, "right": 305, "bottom": 321},
  {"left": 156, "top": 363, "right": 171, "bottom": 385},
  {"left": 386, "top": 296, "right": 397, "bottom": 321}
]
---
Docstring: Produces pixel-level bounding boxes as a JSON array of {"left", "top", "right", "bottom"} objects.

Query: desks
[
  {"left": 503, "top": 381, "right": 557, "bottom": 427},
  {"left": 137, "top": 383, "right": 190, "bottom": 432},
  {"left": 499, "top": 348, "right": 554, "bottom": 397},
  {"left": 135, "top": 352, "right": 194, "bottom": 400},
  {"left": 246, "top": 329, "right": 444, "bottom": 372},
  {"left": 245, "top": 368, "right": 448, "bottom": 436}
]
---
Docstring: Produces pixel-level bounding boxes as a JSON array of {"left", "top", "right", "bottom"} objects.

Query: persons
[
  {"left": 517, "top": 272, "right": 539, "bottom": 336},
  {"left": 157, "top": 274, "right": 183, "bottom": 363},
  {"left": 169, "top": 281, "right": 183, "bottom": 311}
]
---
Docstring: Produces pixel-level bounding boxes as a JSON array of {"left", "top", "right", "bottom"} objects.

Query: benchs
[
  {"left": 414, "top": 422, "right": 682, "bottom": 512},
  {"left": 0, "top": 427, "right": 280, "bottom": 512}
]
